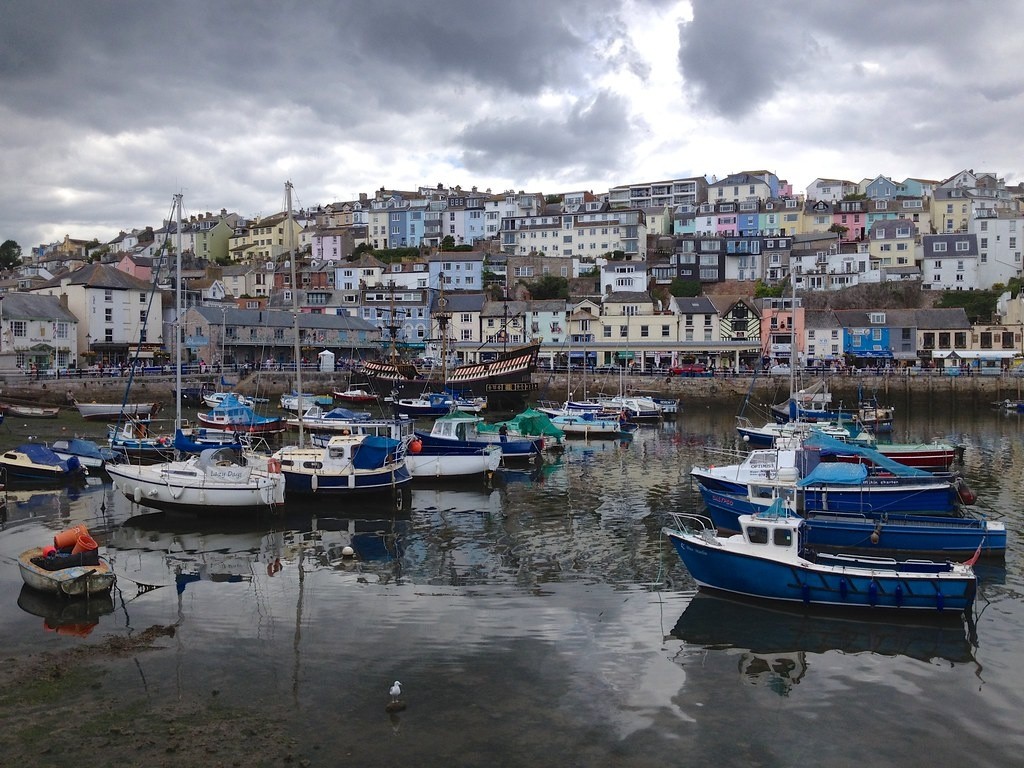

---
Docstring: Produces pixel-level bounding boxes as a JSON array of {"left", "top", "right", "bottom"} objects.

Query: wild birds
[{"left": 390, "top": 681, "right": 403, "bottom": 703}]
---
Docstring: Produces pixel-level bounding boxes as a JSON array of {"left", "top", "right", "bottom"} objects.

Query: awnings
[
  {"left": 848, "top": 350, "right": 894, "bottom": 359},
  {"left": 616, "top": 352, "right": 635, "bottom": 359}
]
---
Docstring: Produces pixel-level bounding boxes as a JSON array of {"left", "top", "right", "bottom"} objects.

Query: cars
[{"left": 596, "top": 363, "right": 620, "bottom": 374}]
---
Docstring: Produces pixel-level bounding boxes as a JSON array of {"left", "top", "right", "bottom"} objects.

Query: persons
[
  {"left": 301, "top": 357, "right": 310, "bottom": 371},
  {"left": 243, "top": 357, "right": 277, "bottom": 374},
  {"left": 94, "top": 360, "right": 145, "bottom": 376},
  {"left": 66, "top": 390, "right": 74, "bottom": 406},
  {"left": 499, "top": 423, "right": 508, "bottom": 442},
  {"left": 200, "top": 360, "right": 207, "bottom": 374},
  {"left": 335, "top": 356, "right": 355, "bottom": 370}
]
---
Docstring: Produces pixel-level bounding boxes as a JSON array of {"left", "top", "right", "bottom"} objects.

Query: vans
[{"left": 669, "top": 363, "right": 707, "bottom": 377}]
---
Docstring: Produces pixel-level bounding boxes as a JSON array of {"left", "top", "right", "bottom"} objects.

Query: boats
[
  {"left": 535, "top": 389, "right": 680, "bottom": 435},
  {"left": 414, "top": 417, "right": 542, "bottom": 457},
  {"left": 770, "top": 400, "right": 895, "bottom": 433},
  {"left": 667, "top": 590, "right": 979, "bottom": 698},
  {"left": 197, "top": 312, "right": 288, "bottom": 433},
  {"left": 203, "top": 392, "right": 253, "bottom": 411},
  {"left": 50, "top": 439, "right": 124, "bottom": 470},
  {"left": 17, "top": 585, "right": 117, "bottom": 638},
  {"left": 279, "top": 392, "right": 333, "bottom": 413},
  {"left": 392, "top": 391, "right": 480, "bottom": 415},
  {"left": 330, "top": 388, "right": 379, "bottom": 402},
  {"left": 86, "top": 511, "right": 287, "bottom": 588},
  {"left": 989, "top": 399, "right": 1024, "bottom": 412},
  {"left": 411, "top": 481, "right": 506, "bottom": 521},
  {"left": 104, "top": 447, "right": 286, "bottom": 515},
  {"left": 664, "top": 498, "right": 980, "bottom": 615},
  {"left": 0, "top": 443, "right": 89, "bottom": 480},
  {"left": 802, "top": 509, "right": 1008, "bottom": 553},
  {"left": 8, "top": 402, "right": 61, "bottom": 419},
  {"left": 309, "top": 412, "right": 502, "bottom": 482},
  {"left": 286, "top": 405, "right": 354, "bottom": 432},
  {"left": 15, "top": 546, "right": 116, "bottom": 597},
  {"left": 73, "top": 401, "right": 158, "bottom": 421},
  {"left": 687, "top": 448, "right": 960, "bottom": 534},
  {"left": 772, "top": 439, "right": 958, "bottom": 471}
]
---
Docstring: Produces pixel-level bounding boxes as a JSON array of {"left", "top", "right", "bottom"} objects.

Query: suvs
[{"left": 630, "top": 361, "right": 661, "bottom": 375}]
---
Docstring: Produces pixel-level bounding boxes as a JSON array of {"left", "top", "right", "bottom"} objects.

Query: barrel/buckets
[
  {"left": 53, "top": 523, "right": 90, "bottom": 549},
  {"left": 71, "top": 535, "right": 97, "bottom": 557}
]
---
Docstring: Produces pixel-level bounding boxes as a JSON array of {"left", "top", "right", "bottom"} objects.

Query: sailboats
[
  {"left": 734, "top": 262, "right": 876, "bottom": 450},
  {"left": 237, "top": 180, "right": 413, "bottom": 495},
  {"left": 104, "top": 192, "right": 249, "bottom": 461},
  {"left": 348, "top": 237, "right": 542, "bottom": 424}
]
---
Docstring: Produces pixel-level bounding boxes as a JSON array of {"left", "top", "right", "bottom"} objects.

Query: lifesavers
[
  {"left": 266, "top": 561, "right": 282, "bottom": 577},
  {"left": 620, "top": 411, "right": 625, "bottom": 419},
  {"left": 162, "top": 437, "right": 173, "bottom": 448},
  {"left": 267, "top": 457, "right": 281, "bottom": 473}
]
